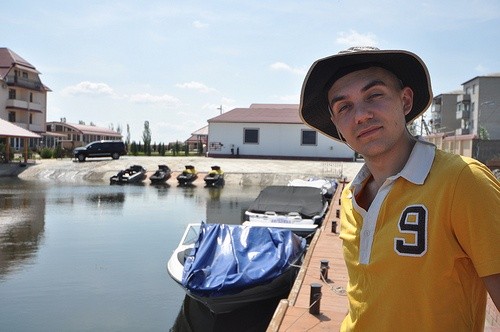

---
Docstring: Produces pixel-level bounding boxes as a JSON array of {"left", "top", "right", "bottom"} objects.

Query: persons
[{"left": 299, "top": 45, "right": 496, "bottom": 332}]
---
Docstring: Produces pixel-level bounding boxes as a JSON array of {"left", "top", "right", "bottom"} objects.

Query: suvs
[{"left": 72, "top": 139, "right": 127, "bottom": 162}]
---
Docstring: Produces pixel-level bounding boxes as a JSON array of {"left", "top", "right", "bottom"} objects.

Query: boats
[
  {"left": 288, "top": 176, "right": 338, "bottom": 199},
  {"left": 242, "top": 184, "right": 329, "bottom": 235},
  {"left": 165, "top": 223, "right": 307, "bottom": 313},
  {"left": 175, "top": 164, "right": 199, "bottom": 183},
  {"left": 203, "top": 166, "right": 224, "bottom": 184},
  {"left": 149, "top": 164, "right": 172, "bottom": 184},
  {"left": 109, "top": 164, "right": 146, "bottom": 185}
]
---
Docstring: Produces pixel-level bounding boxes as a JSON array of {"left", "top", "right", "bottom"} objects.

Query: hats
[{"left": 299, "top": 47, "right": 433, "bottom": 142}]
